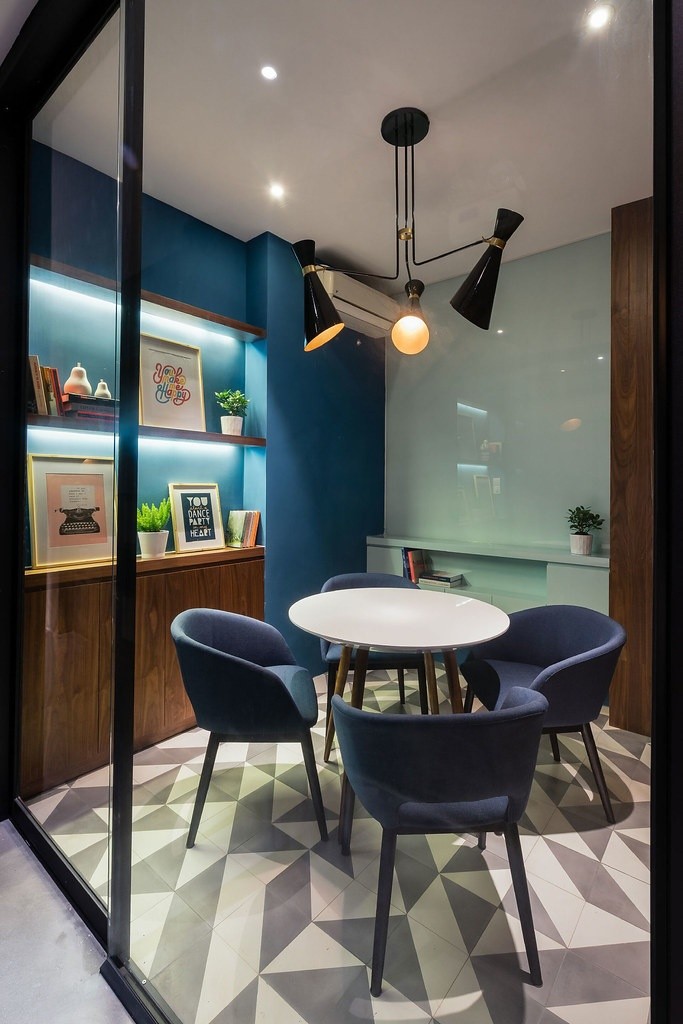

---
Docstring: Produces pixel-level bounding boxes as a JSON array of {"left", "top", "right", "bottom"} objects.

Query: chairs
[
  {"left": 318, "top": 570, "right": 427, "bottom": 742},
  {"left": 458, "top": 604, "right": 626, "bottom": 824},
  {"left": 170, "top": 607, "right": 332, "bottom": 849},
  {"left": 330, "top": 685, "right": 549, "bottom": 997}
]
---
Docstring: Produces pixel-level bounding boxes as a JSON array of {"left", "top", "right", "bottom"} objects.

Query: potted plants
[
  {"left": 214, "top": 387, "right": 251, "bottom": 437},
  {"left": 564, "top": 503, "right": 604, "bottom": 556},
  {"left": 137, "top": 497, "right": 172, "bottom": 559}
]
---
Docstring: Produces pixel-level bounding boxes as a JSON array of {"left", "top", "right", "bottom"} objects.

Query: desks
[{"left": 289, "top": 587, "right": 511, "bottom": 761}]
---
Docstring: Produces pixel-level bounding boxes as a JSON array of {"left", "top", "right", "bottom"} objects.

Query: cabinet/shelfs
[
  {"left": 25, "top": 252, "right": 265, "bottom": 589},
  {"left": 19, "top": 559, "right": 265, "bottom": 802},
  {"left": 366, "top": 533, "right": 612, "bottom": 617}
]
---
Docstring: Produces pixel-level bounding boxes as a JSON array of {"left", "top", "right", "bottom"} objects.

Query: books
[
  {"left": 224, "top": 510, "right": 260, "bottom": 548},
  {"left": 28, "top": 355, "right": 120, "bottom": 422},
  {"left": 419, "top": 578, "right": 462, "bottom": 588},
  {"left": 401, "top": 548, "right": 426, "bottom": 584},
  {"left": 419, "top": 570, "right": 461, "bottom": 582}
]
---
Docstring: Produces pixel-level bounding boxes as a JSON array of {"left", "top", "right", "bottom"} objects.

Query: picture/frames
[
  {"left": 27, "top": 453, "right": 117, "bottom": 569},
  {"left": 139, "top": 331, "right": 207, "bottom": 432},
  {"left": 169, "top": 483, "right": 226, "bottom": 553}
]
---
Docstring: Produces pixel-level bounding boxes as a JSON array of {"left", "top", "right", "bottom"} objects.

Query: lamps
[{"left": 288, "top": 106, "right": 525, "bottom": 355}]
